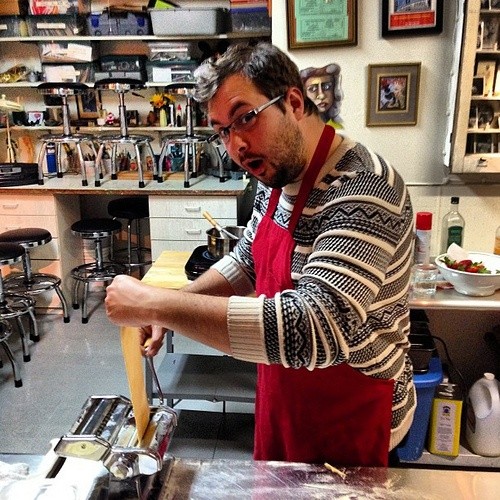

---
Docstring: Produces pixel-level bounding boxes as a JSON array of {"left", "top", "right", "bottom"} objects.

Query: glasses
[{"left": 208, "top": 92, "right": 285, "bottom": 148}]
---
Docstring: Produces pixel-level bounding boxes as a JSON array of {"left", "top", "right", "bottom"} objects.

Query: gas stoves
[{"left": 185, "top": 245, "right": 219, "bottom": 280}]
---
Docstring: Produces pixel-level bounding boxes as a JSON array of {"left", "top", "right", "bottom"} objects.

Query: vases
[{"left": 160, "top": 107, "right": 168, "bottom": 127}]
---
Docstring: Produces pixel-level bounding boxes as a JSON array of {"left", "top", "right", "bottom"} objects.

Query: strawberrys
[{"left": 443, "top": 256, "right": 492, "bottom": 273}]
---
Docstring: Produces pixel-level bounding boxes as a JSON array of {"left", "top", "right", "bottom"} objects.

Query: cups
[
  {"left": 103, "top": 159, "right": 112, "bottom": 173},
  {"left": 85, "top": 160, "right": 95, "bottom": 177},
  {"left": 170, "top": 152, "right": 185, "bottom": 171},
  {"left": 412, "top": 264, "right": 439, "bottom": 296}
]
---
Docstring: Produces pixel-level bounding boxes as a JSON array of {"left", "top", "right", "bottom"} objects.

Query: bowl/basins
[{"left": 435, "top": 251, "right": 500, "bottom": 296}]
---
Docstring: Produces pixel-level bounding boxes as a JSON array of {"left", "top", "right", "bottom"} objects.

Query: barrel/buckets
[{"left": 428, "top": 379, "right": 463, "bottom": 457}]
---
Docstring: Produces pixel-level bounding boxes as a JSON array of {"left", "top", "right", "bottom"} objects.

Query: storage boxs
[
  {"left": 230, "top": 7, "right": 270, "bottom": 33},
  {"left": 148, "top": 7, "right": 223, "bottom": 35},
  {"left": 397, "top": 326, "right": 443, "bottom": 462}
]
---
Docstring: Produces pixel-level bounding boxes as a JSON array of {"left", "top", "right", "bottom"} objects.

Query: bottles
[
  {"left": 46, "top": 144, "right": 56, "bottom": 172},
  {"left": 440, "top": 197, "right": 466, "bottom": 255},
  {"left": 414, "top": 212, "right": 432, "bottom": 265},
  {"left": 464, "top": 372, "right": 500, "bottom": 457},
  {"left": 493, "top": 226, "right": 500, "bottom": 256}
]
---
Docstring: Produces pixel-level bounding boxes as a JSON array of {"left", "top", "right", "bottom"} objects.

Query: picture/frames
[
  {"left": 381, "top": 0, "right": 443, "bottom": 39},
  {"left": 364, "top": 61, "right": 422, "bottom": 128},
  {"left": 285, "top": 0, "right": 359, "bottom": 51},
  {"left": 73, "top": 89, "right": 102, "bottom": 120}
]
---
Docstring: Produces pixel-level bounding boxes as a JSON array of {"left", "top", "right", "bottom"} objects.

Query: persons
[{"left": 104, "top": 41, "right": 414, "bottom": 469}]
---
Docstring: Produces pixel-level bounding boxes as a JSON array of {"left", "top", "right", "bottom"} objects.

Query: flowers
[{"left": 150, "top": 92, "right": 181, "bottom": 108}]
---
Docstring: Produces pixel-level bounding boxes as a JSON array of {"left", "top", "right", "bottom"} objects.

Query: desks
[{"left": 406, "top": 288, "right": 500, "bottom": 312}]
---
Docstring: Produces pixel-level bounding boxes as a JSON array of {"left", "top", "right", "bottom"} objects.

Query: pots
[{"left": 205, "top": 226, "right": 246, "bottom": 259}]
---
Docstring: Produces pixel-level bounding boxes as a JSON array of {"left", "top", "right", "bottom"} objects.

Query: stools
[
  {"left": 107, "top": 198, "right": 149, "bottom": 276},
  {"left": 38, "top": 83, "right": 89, "bottom": 186},
  {"left": 158, "top": 83, "right": 225, "bottom": 187},
  {"left": 94, "top": 78, "right": 158, "bottom": 188},
  {"left": 0, "top": 228, "right": 70, "bottom": 388},
  {"left": 70, "top": 217, "right": 128, "bottom": 324}
]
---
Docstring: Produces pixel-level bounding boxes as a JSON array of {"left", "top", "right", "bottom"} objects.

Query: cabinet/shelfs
[
  {"left": 449, "top": 0, "right": 500, "bottom": 173},
  {"left": 0, "top": 0, "right": 273, "bottom": 317}
]
